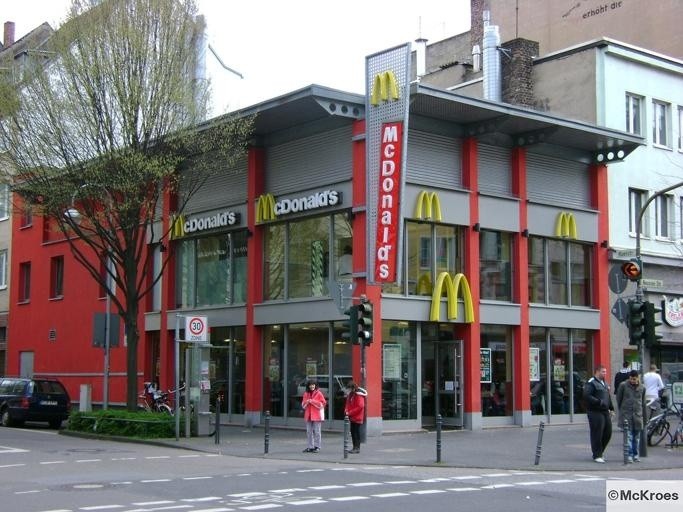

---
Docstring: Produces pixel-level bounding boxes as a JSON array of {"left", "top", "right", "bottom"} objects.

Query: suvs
[{"left": 0, "top": 376, "right": 72, "bottom": 427}]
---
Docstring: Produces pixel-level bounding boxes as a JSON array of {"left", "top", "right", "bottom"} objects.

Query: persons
[
  {"left": 614, "top": 360, "right": 632, "bottom": 433},
  {"left": 643, "top": 364, "right": 665, "bottom": 423},
  {"left": 301, "top": 379, "right": 327, "bottom": 453},
  {"left": 584, "top": 365, "right": 614, "bottom": 463},
  {"left": 616, "top": 370, "right": 646, "bottom": 464},
  {"left": 344, "top": 381, "right": 368, "bottom": 454}
]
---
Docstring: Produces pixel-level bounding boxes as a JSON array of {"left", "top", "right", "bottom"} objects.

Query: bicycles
[
  {"left": 137, "top": 380, "right": 215, "bottom": 437},
  {"left": 645, "top": 384, "right": 682, "bottom": 447}
]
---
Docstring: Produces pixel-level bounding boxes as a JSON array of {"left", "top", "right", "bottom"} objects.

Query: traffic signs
[{"left": 185, "top": 315, "right": 206, "bottom": 342}]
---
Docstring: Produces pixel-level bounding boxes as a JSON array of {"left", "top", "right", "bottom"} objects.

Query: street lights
[{"left": 60, "top": 184, "right": 115, "bottom": 411}]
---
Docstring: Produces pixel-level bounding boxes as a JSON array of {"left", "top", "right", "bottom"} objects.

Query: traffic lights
[
  {"left": 357, "top": 303, "right": 374, "bottom": 345},
  {"left": 340, "top": 304, "right": 360, "bottom": 347},
  {"left": 622, "top": 259, "right": 644, "bottom": 281},
  {"left": 642, "top": 301, "right": 664, "bottom": 350},
  {"left": 626, "top": 299, "right": 645, "bottom": 346}
]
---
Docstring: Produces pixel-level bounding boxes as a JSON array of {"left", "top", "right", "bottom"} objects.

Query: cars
[
  {"left": 213, "top": 373, "right": 414, "bottom": 421},
  {"left": 474, "top": 370, "right": 587, "bottom": 416}
]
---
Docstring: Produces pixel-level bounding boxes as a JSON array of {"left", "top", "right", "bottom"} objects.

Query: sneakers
[
  {"left": 300, "top": 446, "right": 321, "bottom": 454},
  {"left": 590, "top": 453, "right": 642, "bottom": 466},
  {"left": 348, "top": 446, "right": 361, "bottom": 454}
]
